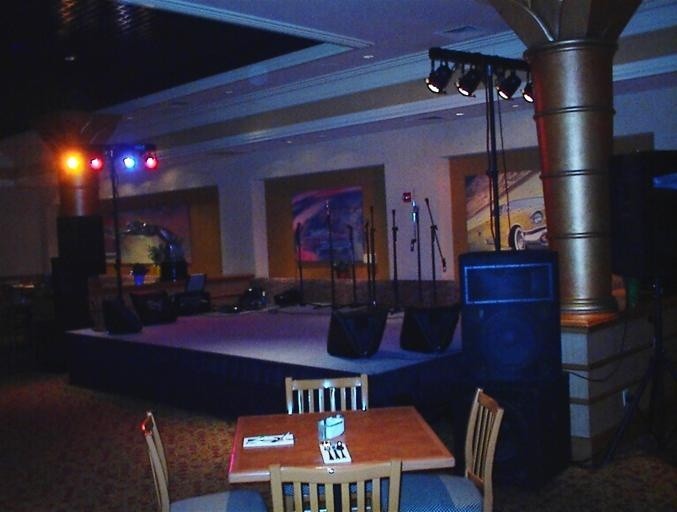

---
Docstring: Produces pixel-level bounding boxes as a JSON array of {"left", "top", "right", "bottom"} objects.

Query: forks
[
  {"left": 323, "top": 439, "right": 346, "bottom": 460},
  {"left": 248, "top": 430, "right": 295, "bottom": 442}
]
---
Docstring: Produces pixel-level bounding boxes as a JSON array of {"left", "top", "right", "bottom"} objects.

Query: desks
[{"left": 225, "top": 402, "right": 457, "bottom": 512}]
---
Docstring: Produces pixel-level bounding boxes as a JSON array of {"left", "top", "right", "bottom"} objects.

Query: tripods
[
  {"left": 601, "top": 279, "right": 677, "bottom": 465},
  {"left": 290, "top": 199, "right": 446, "bottom": 314}
]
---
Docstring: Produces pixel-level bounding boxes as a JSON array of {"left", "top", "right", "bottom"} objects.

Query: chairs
[
  {"left": 266, "top": 456, "right": 403, "bottom": 511},
  {"left": 136, "top": 407, "right": 269, "bottom": 512},
  {"left": 379, "top": 389, "right": 507, "bottom": 512},
  {"left": 282, "top": 373, "right": 371, "bottom": 511}
]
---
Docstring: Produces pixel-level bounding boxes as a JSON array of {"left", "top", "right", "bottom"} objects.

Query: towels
[
  {"left": 317, "top": 439, "right": 351, "bottom": 465},
  {"left": 239, "top": 432, "right": 293, "bottom": 446}
]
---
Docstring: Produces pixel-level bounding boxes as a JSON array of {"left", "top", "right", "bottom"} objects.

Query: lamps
[
  {"left": 60, "top": 141, "right": 158, "bottom": 171},
  {"left": 421, "top": 44, "right": 539, "bottom": 103}
]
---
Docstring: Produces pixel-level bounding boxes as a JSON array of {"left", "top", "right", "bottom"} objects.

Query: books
[{"left": 242, "top": 434, "right": 293, "bottom": 448}]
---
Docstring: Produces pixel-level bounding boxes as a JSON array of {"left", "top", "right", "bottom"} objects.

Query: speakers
[
  {"left": 327, "top": 311, "right": 387, "bottom": 359},
  {"left": 102, "top": 295, "right": 143, "bottom": 333},
  {"left": 400, "top": 303, "right": 461, "bottom": 354},
  {"left": 458, "top": 249, "right": 571, "bottom": 491},
  {"left": 609, "top": 151, "right": 677, "bottom": 279},
  {"left": 130, "top": 289, "right": 176, "bottom": 326},
  {"left": 169, "top": 291, "right": 211, "bottom": 317}
]
